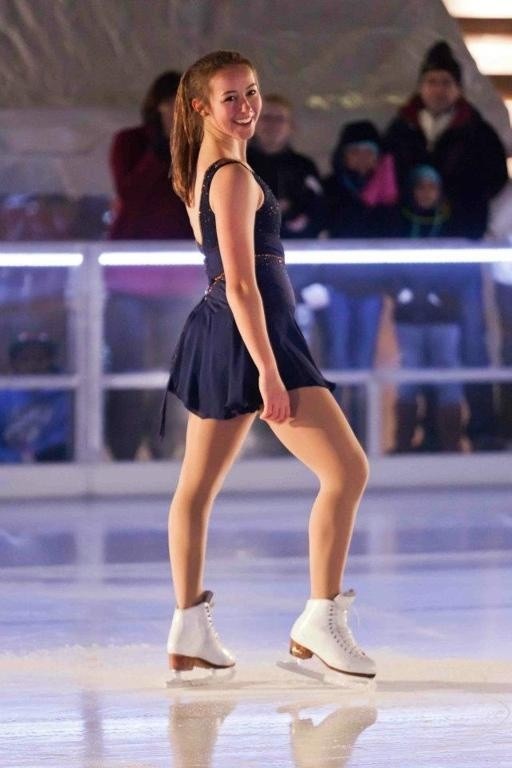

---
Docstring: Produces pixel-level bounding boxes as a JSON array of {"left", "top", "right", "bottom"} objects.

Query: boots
[
  {"left": 167, "top": 590, "right": 236, "bottom": 671},
  {"left": 287, "top": 589, "right": 377, "bottom": 679}
]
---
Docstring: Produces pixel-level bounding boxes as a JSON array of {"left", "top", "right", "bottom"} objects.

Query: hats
[{"left": 420, "top": 40, "right": 461, "bottom": 83}]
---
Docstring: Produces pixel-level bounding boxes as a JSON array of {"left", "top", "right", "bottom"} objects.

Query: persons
[
  {"left": 313, "top": 119, "right": 390, "bottom": 448},
  {"left": 248, "top": 95, "right": 326, "bottom": 453},
  {"left": 379, "top": 166, "right": 464, "bottom": 454},
  {"left": 98, "top": 68, "right": 190, "bottom": 461},
  {"left": 162, "top": 50, "right": 378, "bottom": 683},
  {"left": 484, "top": 171, "right": 511, "bottom": 449},
  {"left": 383, "top": 33, "right": 504, "bottom": 451}
]
